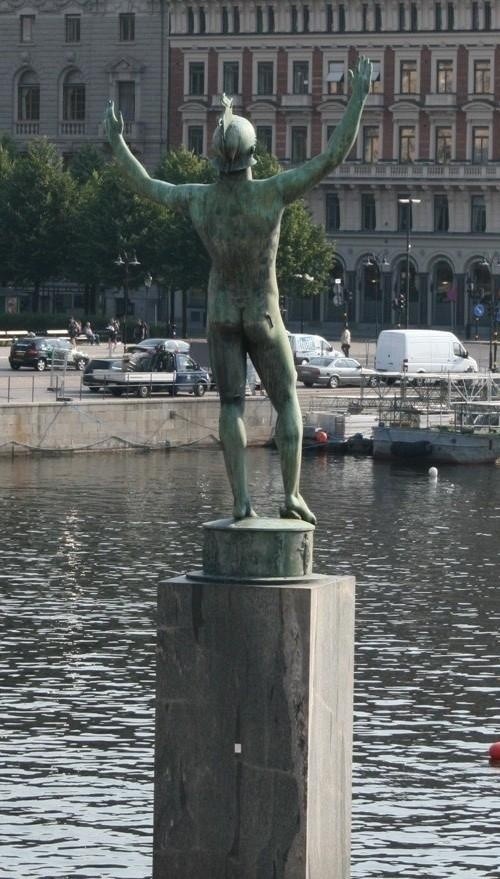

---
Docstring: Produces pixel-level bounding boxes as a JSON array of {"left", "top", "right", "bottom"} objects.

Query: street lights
[
  {"left": 397, "top": 193, "right": 422, "bottom": 328},
  {"left": 113, "top": 246, "right": 141, "bottom": 343},
  {"left": 292, "top": 273, "right": 314, "bottom": 334},
  {"left": 142, "top": 270, "right": 152, "bottom": 340},
  {"left": 478, "top": 249, "right": 500, "bottom": 372},
  {"left": 362, "top": 249, "right": 390, "bottom": 345}
]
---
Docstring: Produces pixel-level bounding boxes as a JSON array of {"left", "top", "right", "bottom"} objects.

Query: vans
[
  {"left": 374, "top": 329, "right": 479, "bottom": 387},
  {"left": 288, "top": 333, "right": 345, "bottom": 366}
]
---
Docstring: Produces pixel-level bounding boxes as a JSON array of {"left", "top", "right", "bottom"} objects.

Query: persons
[
  {"left": 100, "top": 53, "right": 374, "bottom": 524},
  {"left": 340, "top": 325, "right": 351, "bottom": 358},
  {"left": 67, "top": 316, "right": 147, "bottom": 348}
]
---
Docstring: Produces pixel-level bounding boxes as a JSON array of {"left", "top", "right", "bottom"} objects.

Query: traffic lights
[{"left": 399, "top": 292, "right": 405, "bottom": 310}]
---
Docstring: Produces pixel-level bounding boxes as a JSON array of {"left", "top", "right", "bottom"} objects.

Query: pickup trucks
[{"left": 92, "top": 351, "right": 211, "bottom": 398}]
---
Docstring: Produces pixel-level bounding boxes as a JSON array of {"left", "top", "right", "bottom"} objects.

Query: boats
[{"left": 372, "top": 401, "right": 500, "bottom": 465}]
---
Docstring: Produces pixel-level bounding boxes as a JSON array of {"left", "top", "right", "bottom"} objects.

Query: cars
[
  {"left": 83, "top": 358, "right": 137, "bottom": 393},
  {"left": 295, "top": 356, "right": 382, "bottom": 388},
  {"left": 9, "top": 337, "right": 90, "bottom": 372},
  {"left": 126, "top": 339, "right": 190, "bottom": 354}
]
